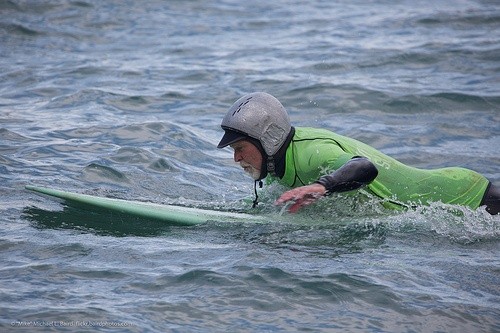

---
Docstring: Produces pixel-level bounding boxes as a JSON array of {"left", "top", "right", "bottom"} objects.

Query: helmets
[{"left": 216, "top": 91, "right": 292, "bottom": 155}]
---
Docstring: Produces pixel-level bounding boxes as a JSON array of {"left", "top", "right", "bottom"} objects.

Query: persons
[{"left": 214, "top": 92, "right": 500, "bottom": 225}]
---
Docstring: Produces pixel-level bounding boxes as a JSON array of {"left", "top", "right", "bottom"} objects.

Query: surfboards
[{"left": 24, "top": 184, "right": 418, "bottom": 231}]
